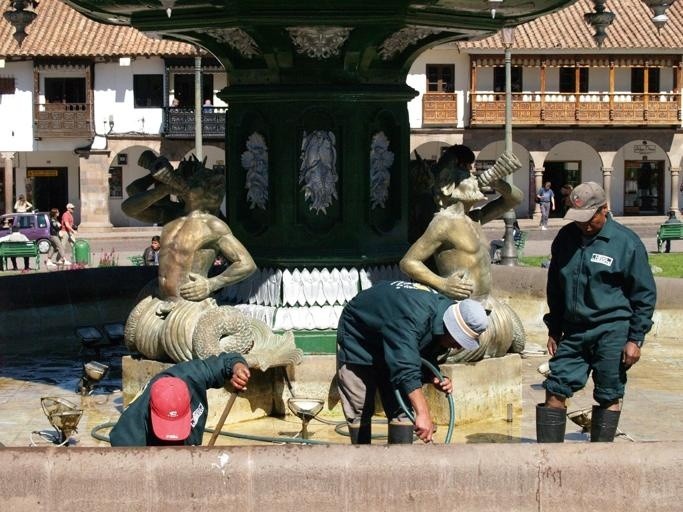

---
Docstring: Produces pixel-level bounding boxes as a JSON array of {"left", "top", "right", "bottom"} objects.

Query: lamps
[
  {"left": 566, "top": 407, "right": 592, "bottom": 434},
  {"left": 287, "top": 397, "right": 324, "bottom": 439},
  {"left": 40, "top": 321, "right": 125, "bottom": 446}
]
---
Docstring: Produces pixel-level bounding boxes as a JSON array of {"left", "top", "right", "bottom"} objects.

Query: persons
[
  {"left": 169, "top": 99, "right": 179, "bottom": 113},
  {"left": 664, "top": 211, "right": 680, "bottom": 254},
  {"left": 438, "top": 143, "right": 476, "bottom": 171},
  {"left": 120, "top": 163, "right": 257, "bottom": 302},
  {"left": 44, "top": 208, "right": 72, "bottom": 267},
  {"left": 124, "top": 169, "right": 171, "bottom": 207},
  {"left": 335, "top": 281, "right": 488, "bottom": 444},
  {"left": 537, "top": 181, "right": 556, "bottom": 231},
  {"left": 0, "top": 226, "right": 32, "bottom": 270},
  {"left": 398, "top": 153, "right": 524, "bottom": 301},
  {"left": 143, "top": 236, "right": 160, "bottom": 267},
  {"left": 108, "top": 351, "right": 251, "bottom": 447},
  {"left": 489, "top": 220, "right": 521, "bottom": 263},
  {"left": 61, "top": 203, "right": 77, "bottom": 261},
  {"left": 560, "top": 181, "right": 573, "bottom": 217},
  {"left": 14, "top": 194, "right": 32, "bottom": 213},
  {"left": 535, "top": 181, "right": 657, "bottom": 441},
  {"left": 202, "top": 99, "right": 213, "bottom": 114}
]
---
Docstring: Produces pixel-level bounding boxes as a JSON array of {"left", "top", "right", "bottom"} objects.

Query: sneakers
[{"left": 46, "top": 259, "right": 72, "bottom": 266}]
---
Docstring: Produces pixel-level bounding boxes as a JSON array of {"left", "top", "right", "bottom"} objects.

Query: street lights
[
  {"left": 500, "top": 27, "right": 517, "bottom": 266},
  {"left": 189, "top": 43, "right": 208, "bottom": 162}
]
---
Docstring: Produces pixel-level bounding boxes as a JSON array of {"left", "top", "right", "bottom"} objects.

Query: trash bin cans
[{"left": 72, "top": 239, "right": 91, "bottom": 264}]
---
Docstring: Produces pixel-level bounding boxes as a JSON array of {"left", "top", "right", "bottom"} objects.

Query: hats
[
  {"left": 66, "top": 203, "right": 75, "bottom": 209},
  {"left": 443, "top": 299, "right": 488, "bottom": 351},
  {"left": 563, "top": 181, "right": 608, "bottom": 223},
  {"left": 149, "top": 376, "right": 191, "bottom": 441}
]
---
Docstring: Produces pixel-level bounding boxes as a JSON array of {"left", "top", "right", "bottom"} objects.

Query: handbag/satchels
[{"left": 534, "top": 197, "right": 541, "bottom": 203}]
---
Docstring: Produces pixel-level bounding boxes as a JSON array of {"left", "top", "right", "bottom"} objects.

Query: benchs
[
  {"left": 657, "top": 223, "right": 683, "bottom": 253},
  {"left": 495, "top": 231, "right": 529, "bottom": 261},
  {"left": 0, "top": 240, "right": 40, "bottom": 271}
]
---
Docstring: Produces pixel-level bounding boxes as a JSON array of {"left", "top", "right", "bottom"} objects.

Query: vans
[{"left": 0, "top": 212, "right": 52, "bottom": 254}]
